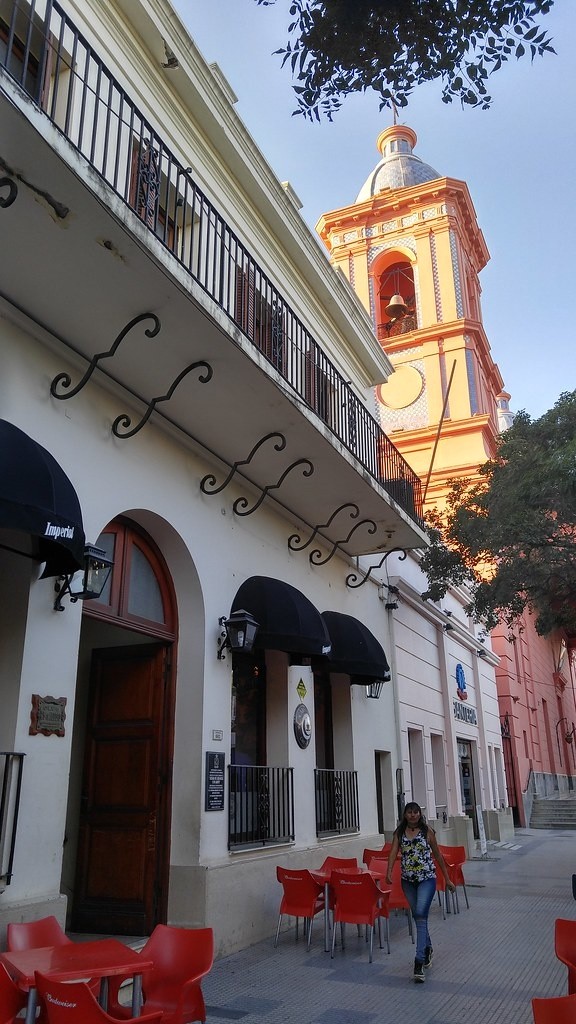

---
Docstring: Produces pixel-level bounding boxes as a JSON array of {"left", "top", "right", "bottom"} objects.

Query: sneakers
[
  {"left": 423, "top": 946, "right": 434, "bottom": 967},
  {"left": 414, "top": 957, "right": 425, "bottom": 982}
]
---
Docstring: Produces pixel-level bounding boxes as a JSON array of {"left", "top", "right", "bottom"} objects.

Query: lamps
[
  {"left": 217, "top": 609, "right": 259, "bottom": 660},
  {"left": 443, "top": 609, "right": 452, "bottom": 617},
  {"left": 387, "top": 603, "right": 399, "bottom": 609},
  {"left": 158, "top": 35, "right": 179, "bottom": 70},
  {"left": 54, "top": 542, "right": 115, "bottom": 612},
  {"left": 382, "top": 581, "right": 399, "bottom": 595},
  {"left": 366, "top": 673, "right": 391, "bottom": 699},
  {"left": 477, "top": 637, "right": 485, "bottom": 643},
  {"left": 477, "top": 650, "right": 487, "bottom": 657},
  {"left": 443, "top": 624, "right": 454, "bottom": 631},
  {"left": 556, "top": 717, "right": 573, "bottom": 744}
]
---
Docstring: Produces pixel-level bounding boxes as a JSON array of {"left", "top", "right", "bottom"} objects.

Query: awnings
[
  {"left": 231, "top": 576, "right": 331, "bottom": 666},
  {"left": 0, "top": 419, "right": 85, "bottom": 579},
  {"left": 320, "top": 611, "right": 390, "bottom": 686}
]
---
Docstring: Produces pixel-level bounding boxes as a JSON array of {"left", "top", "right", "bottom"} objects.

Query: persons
[{"left": 385, "top": 802, "right": 455, "bottom": 983}]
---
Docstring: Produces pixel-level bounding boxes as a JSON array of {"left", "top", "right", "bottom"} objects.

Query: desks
[
  {"left": 0, "top": 939, "right": 153, "bottom": 1024},
  {"left": 308, "top": 868, "right": 385, "bottom": 952}
]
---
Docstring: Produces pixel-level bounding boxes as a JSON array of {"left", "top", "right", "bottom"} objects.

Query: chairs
[
  {"left": 532, "top": 919, "right": 576, "bottom": 1024},
  {"left": 0, "top": 916, "right": 213, "bottom": 1024},
  {"left": 273, "top": 842, "right": 469, "bottom": 963}
]
